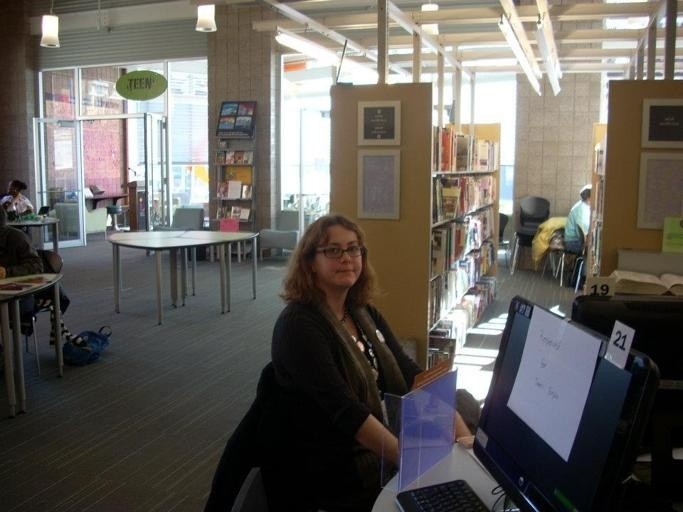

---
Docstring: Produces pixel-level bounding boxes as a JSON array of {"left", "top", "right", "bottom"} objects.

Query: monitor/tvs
[
  {"left": 572, "top": 294, "right": 683, "bottom": 502},
  {"left": 472, "top": 294, "right": 660, "bottom": 512}
]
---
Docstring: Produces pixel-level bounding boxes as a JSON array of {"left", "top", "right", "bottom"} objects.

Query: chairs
[
  {"left": 51, "top": 203, "right": 108, "bottom": 238},
  {"left": 499, "top": 197, "right": 587, "bottom": 295},
  {"left": 256, "top": 210, "right": 306, "bottom": 259},
  {"left": 161, "top": 207, "right": 204, "bottom": 230}
]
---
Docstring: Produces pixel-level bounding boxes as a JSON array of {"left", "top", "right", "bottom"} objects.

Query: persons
[
  {"left": 272, "top": 214, "right": 480, "bottom": 512},
  {"left": 563, "top": 184, "right": 595, "bottom": 290},
  {"left": 0, "top": 180, "right": 34, "bottom": 220},
  {"left": 0, "top": 204, "right": 43, "bottom": 324}
]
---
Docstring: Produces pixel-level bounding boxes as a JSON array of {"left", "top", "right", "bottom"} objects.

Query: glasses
[{"left": 313, "top": 244, "right": 364, "bottom": 260}]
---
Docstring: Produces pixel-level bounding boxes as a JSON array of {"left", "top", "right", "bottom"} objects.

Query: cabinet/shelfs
[
  {"left": 586, "top": 76, "right": 682, "bottom": 283},
  {"left": 326, "top": 81, "right": 502, "bottom": 367}
]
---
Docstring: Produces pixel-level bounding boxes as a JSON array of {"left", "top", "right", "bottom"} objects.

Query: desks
[
  {"left": 106, "top": 228, "right": 259, "bottom": 323},
  {"left": 8, "top": 213, "right": 58, "bottom": 258},
  {"left": 373, "top": 432, "right": 682, "bottom": 512},
  {"left": 0, "top": 272, "right": 63, "bottom": 416}
]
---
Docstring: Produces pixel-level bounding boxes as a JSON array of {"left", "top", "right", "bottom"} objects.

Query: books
[
  {"left": 427, "top": 123, "right": 499, "bottom": 373},
  {"left": 216, "top": 100, "right": 257, "bottom": 223},
  {"left": 609, "top": 269, "right": 683, "bottom": 296},
  {"left": 590, "top": 139, "right": 605, "bottom": 277}
]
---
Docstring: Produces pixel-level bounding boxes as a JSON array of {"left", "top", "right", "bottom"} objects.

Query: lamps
[
  {"left": 37, "top": 0, "right": 65, "bottom": 48},
  {"left": 194, "top": 2, "right": 218, "bottom": 34}
]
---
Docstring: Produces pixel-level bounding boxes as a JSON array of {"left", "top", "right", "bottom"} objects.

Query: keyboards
[{"left": 395, "top": 479, "right": 490, "bottom": 512}]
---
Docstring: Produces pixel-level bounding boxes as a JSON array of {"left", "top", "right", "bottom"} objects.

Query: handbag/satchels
[{"left": 60, "top": 324, "right": 115, "bottom": 365}]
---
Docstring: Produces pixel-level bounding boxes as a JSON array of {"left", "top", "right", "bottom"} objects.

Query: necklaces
[{"left": 341, "top": 307, "right": 349, "bottom": 322}]
